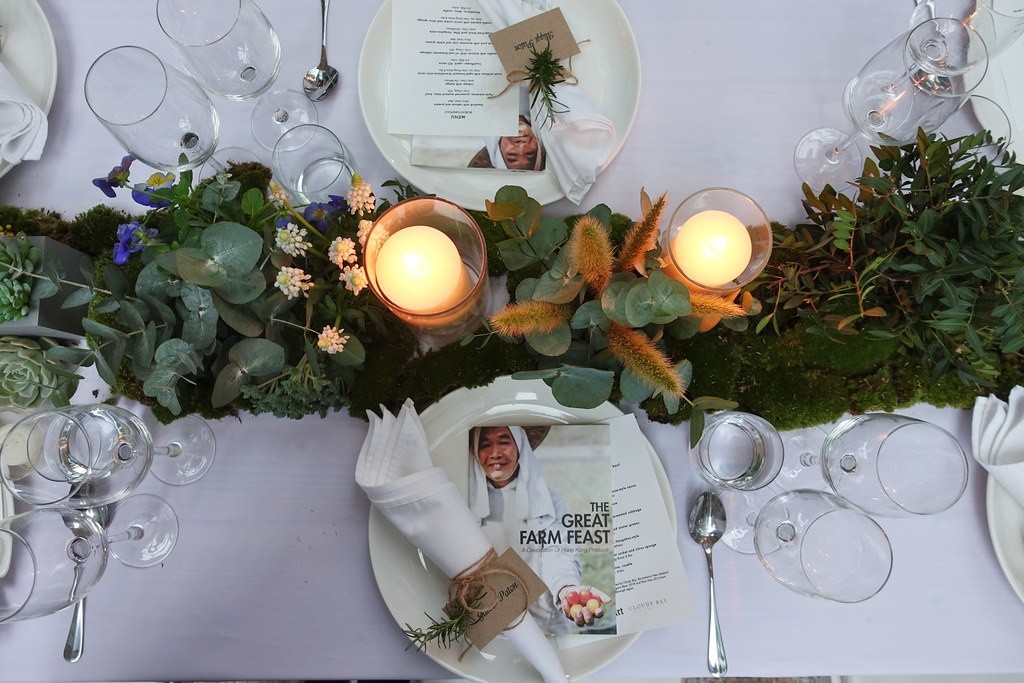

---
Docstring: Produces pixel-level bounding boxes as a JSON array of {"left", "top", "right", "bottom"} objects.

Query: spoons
[
  {"left": 63, "top": 482, "right": 110, "bottom": 663},
  {"left": 303, "top": 0, "right": 339, "bottom": 102},
  {"left": 688, "top": 491, "right": 727, "bottom": 678}
]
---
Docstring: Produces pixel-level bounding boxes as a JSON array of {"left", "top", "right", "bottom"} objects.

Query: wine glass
[
  {"left": 156, "top": 0, "right": 318, "bottom": 153},
  {"left": 84, "top": 46, "right": 263, "bottom": 183},
  {"left": 0, "top": 403, "right": 216, "bottom": 509},
  {"left": 793, "top": 17, "right": 989, "bottom": 192},
  {"left": 0, "top": 494, "right": 179, "bottom": 625},
  {"left": 716, "top": 487, "right": 893, "bottom": 603},
  {"left": 766, "top": 412, "right": 969, "bottom": 518},
  {"left": 842, "top": 0, "right": 1024, "bottom": 146}
]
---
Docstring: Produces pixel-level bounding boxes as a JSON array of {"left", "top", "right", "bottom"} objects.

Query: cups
[
  {"left": 272, "top": 124, "right": 355, "bottom": 205},
  {"left": 900, "top": 95, "right": 1011, "bottom": 170},
  {"left": 688, "top": 411, "right": 784, "bottom": 492},
  {"left": 658, "top": 186, "right": 773, "bottom": 298},
  {"left": 363, "top": 196, "right": 492, "bottom": 348}
]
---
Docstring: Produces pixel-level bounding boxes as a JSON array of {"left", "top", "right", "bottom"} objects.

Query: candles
[
  {"left": 0, "top": 424, "right": 40, "bottom": 466},
  {"left": 662, "top": 210, "right": 752, "bottom": 292},
  {"left": 375, "top": 225, "right": 474, "bottom": 330}
]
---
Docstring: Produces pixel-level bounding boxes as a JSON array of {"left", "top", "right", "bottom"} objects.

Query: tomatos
[{"left": 566, "top": 589, "right": 602, "bottom": 619}]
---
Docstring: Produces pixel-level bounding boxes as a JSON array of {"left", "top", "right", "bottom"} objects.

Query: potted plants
[{"left": 0, "top": 224, "right": 87, "bottom": 409}]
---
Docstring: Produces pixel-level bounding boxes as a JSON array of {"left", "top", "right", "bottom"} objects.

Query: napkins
[
  {"left": 971, "top": 385, "right": 1024, "bottom": 507},
  {"left": 0, "top": 58, "right": 48, "bottom": 166},
  {"left": 480, "top": 0, "right": 616, "bottom": 205},
  {"left": 355, "top": 399, "right": 572, "bottom": 683}
]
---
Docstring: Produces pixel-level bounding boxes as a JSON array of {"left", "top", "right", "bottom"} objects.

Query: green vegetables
[
  {"left": 524, "top": 40, "right": 571, "bottom": 129},
  {"left": 402, "top": 585, "right": 488, "bottom": 654}
]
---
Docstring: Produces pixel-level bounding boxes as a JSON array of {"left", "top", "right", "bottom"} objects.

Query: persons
[
  {"left": 467, "top": 424, "right": 612, "bottom": 628},
  {"left": 484, "top": 82, "right": 547, "bottom": 171}
]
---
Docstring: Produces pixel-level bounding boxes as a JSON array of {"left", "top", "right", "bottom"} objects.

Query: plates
[
  {"left": 986, "top": 472, "right": 1024, "bottom": 602},
  {"left": 962, "top": 0, "right": 1024, "bottom": 167},
  {"left": 0, "top": 0, "right": 57, "bottom": 179},
  {"left": 358, "top": 0, "right": 642, "bottom": 212},
  {"left": 369, "top": 375, "right": 677, "bottom": 683}
]
